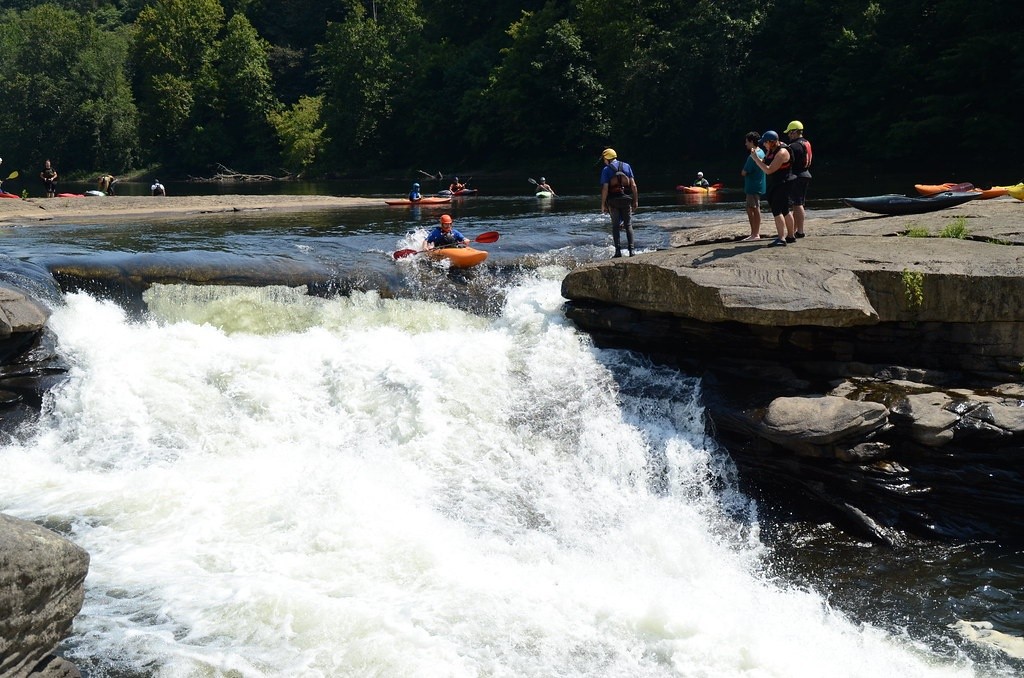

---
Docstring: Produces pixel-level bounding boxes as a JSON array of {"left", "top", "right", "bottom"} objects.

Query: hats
[
  {"left": 783, "top": 120, "right": 804, "bottom": 133},
  {"left": 758, "top": 130, "right": 778, "bottom": 143}
]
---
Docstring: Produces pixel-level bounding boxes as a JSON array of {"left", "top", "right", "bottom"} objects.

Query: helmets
[
  {"left": 413, "top": 183, "right": 421, "bottom": 190},
  {"left": 540, "top": 177, "right": 546, "bottom": 180},
  {"left": 602, "top": 148, "right": 617, "bottom": 160},
  {"left": 440, "top": 214, "right": 452, "bottom": 224},
  {"left": 698, "top": 172, "right": 703, "bottom": 176},
  {"left": 154, "top": 179, "right": 159, "bottom": 184}
]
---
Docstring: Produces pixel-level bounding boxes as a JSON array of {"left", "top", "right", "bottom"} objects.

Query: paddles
[
  {"left": 451, "top": 176, "right": 473, "bottom": 196},
  {"left": 528, "top": 178, "right": 559, "bottom": 197},
  {"left": 917, "top": 182, "right": 974, "bottom": 198},
  {"left": 393, "top": 231, "right": 500, "bottom": 260},
  {"left": 676, "top": 183, "right": 723, "bottom": 190}
]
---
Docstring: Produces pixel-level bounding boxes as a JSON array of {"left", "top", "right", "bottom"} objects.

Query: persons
[
  {"left": 750, "top": 130, "right": 797, "bottom": 246},
  {"left": 692, "top": 171, "right": 709, "bottom": 186},
  {"left": 534, "top": 176, "right": 554, "bottom": 195},
  {"left": 151, "top": 178, "right": 166, "bottom": 196},
  {"left": 422, "top": 215, "right": 470, "bottom": 252},
  {"left": 741, "top": 132, "right": 766, "bottom": 241},
  {"left": 410, "top": 183, "right": 421, "bottom": 201},
  {"left": 600, "top": 148, "right": 638, "bottom": 258},
  {"left": 40, "top": 159, "right": 57, "bottom": 198},
  {"left": 97, "top": 174, "right": 114, "bottom": 196},
  {"left": 784, "top": 120, "right": 812, "bottom": 239},
  {"left": 449, "top": 177, "right": 465, "bottom": 195}
]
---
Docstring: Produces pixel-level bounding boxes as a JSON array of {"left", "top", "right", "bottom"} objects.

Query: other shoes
[
  {"left": 741, "top": 235, "right": 760, "bottom": 241},
  {"left": 768, "top": 239, "right": 786, "bottom": 247},
  {"left": 785, "top": 236, "right": 796, "bottom": 243},
  {"left": 795, "top": 231, "right": 805, "bottom": 238},
  {"left": 630, "top": 252, "right": 635, "bottom": 257},
  {"left": 612, "top": 254, "right": 621, "bottom": 258}
]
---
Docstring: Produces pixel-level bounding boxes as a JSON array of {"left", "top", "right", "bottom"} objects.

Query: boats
[
  {"left": 683, "top": 182, "right": 722, "bottom": 194},
  {"left": 535, "top": 191, "right": 552, "bottom": 198},
  {"left": 0, "top": 192, "right": 19, "bottom": 198},
  {"left": 83, "top": 190, "right": 107, "bottom": 196},
  {"left": 1006, "top": 182, "right": 1024, "bottom": 203},
  {"left": 57, "top": 193, "right": 85, "bottom": 198},
  {"left": 438, "top": 188, "right": 478, "bottom": 195},
  {"left": 837, "top": 190, "right": 983, "bottom": 214},
  {"left": 427, "top": 246, "right": 489, "bottom": 268},
  {"left": 912, "top": 182, "right": 1008, "bottom": 201},
  {"left": 384, "top": 197, "right": 451, "bottom": 205}
]
